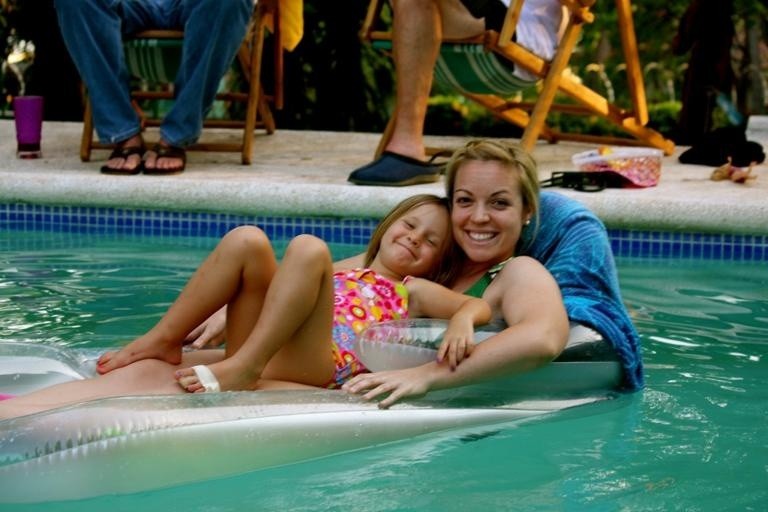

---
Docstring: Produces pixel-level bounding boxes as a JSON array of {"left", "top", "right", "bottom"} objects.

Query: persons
[
  {"left": 668, "top": 0, "right": 733, "bottom": 146},
  {"left": 95, "top": 192, "right": 495, "bottom": 395},
  {"left": 348, "top": 0, "right": 579, "bottom": 186},
  {"left": 182, "top": 138, "right": 571, "bottom": 409},
  {"left": 55, "top": 0, "right": 259, "bottom": 174}
]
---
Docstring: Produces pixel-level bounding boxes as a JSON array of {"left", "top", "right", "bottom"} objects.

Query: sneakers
[{"left": 346, "top": 150, "right": 448, "bottom": 184}]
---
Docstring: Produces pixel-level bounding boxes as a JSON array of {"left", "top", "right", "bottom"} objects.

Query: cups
[{"left": 11, "top": 95, "right": 43, "bottom": 161}]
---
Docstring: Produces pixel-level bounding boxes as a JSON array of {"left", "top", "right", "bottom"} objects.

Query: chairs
[
  {"left": 360, "top": 2, "right": 675, "bottom": 161},
  {"left": 78, "top": 2, "right": 289, "bottom": 166}
]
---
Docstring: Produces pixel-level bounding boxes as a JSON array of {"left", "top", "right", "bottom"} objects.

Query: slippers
[{"left": 100, "top": 141, "right": 186, "bottom": 176}]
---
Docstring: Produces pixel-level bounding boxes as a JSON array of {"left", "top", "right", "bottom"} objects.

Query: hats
[
  {"left": 678, "top": 124, "right": 765, "bottom": 167},
  {"left": 549, "top": 145, "right": 664, "bottom": 192}
]
